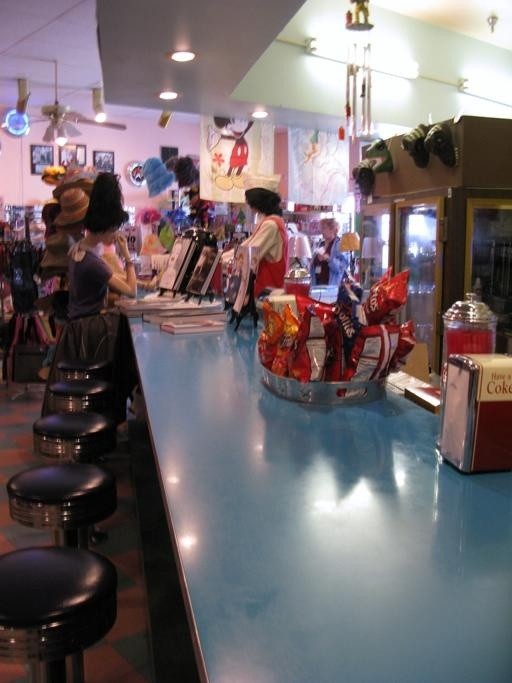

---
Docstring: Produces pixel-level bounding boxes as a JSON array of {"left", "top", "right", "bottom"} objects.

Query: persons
[
  {"left": 223, "top": 173, "right": 288, "bottom": 298},
  {"left": 308, "top": 216, "right": 350, "bottom": 285},
  {"left": 41, "top": 173, "right": 139, "bottom": 455}
]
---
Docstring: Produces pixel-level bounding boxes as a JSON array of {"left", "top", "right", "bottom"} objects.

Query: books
[
  {"left": 142, "top": 311, "right": 228, "bottom": 324},
  {"left": 186, "top": 244, "right": 222, "bottom": 297},
  {"left": 224, "top": 247, "right": 262, "bottom": 306},
  {"left": 159, "top": 236, "right": 196, "bottom": 290},
  {"left": 160, "top": 319, "right": 224, "bottom": 334}
]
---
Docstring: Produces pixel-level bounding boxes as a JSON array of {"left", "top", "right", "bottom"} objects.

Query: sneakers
[
  {"left": 129, "top": 385, "right": 145, "bottom": 415},
  {"left": 116, "top": 422, "right": 131, "bottom": 444},
  {"left": 425, "top": 124, "right": 459, "bottom": 168},
  {"left": 400, "top": 123, "right": 428, "bottom": 168}
]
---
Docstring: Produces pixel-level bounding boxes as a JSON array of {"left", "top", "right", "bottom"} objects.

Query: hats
[
  {"left": 40, "top": 198, "right": 62, "bottom": 238},
  {"left": 54, "top": 187, "right": 90, "bottom": 224},
  {"left": 245, "top": 187, "right": 283, "bottom": 216},
  {"left": 52, "top": 162, "right": 98, "bottom": 200},
  {"left": 40, "top": 232, "right": 77, "bottom": 267}
]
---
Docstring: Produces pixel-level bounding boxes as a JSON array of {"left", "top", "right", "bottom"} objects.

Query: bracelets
[{"left": 121, "top": 256, "right": 135, "bottom": 271}]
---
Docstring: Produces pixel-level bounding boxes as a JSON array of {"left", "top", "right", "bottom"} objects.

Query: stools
[{"left": 1, "top": 357, "right": 119, "bottom": 683}]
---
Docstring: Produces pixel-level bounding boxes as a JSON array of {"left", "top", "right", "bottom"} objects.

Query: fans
[{"left": 1, "top": 59, "right": 127, "bottom": 142}]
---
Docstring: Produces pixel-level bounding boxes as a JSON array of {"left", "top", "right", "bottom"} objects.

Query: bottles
[
  {"left": 440, "top": 293, "right": 498, "bottom": 406},
  {"left": 282, "top": 268, "right": 313, "bottom": 297}
]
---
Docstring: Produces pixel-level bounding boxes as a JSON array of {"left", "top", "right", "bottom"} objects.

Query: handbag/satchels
[{"left": 4, "top": 344, "right": 55, "bottom": 387}]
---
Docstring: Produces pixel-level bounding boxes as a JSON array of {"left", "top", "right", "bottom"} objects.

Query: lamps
[
  {"left": 56, "top": 125, "right": 67, "bottom": 145},
  {"left": 158, "top": 110, "right": 172, "bottom": 126},
  {"left": 92, "top": 87, "right": 107, "bottom": 122},
  {"left": 18, "top": 78, "right": 31, "bottom": 114}
]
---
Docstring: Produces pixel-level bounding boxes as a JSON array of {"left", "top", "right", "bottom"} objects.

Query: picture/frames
[{"left": 30, "top": 144, "right": 114, "bottom": 176}]
